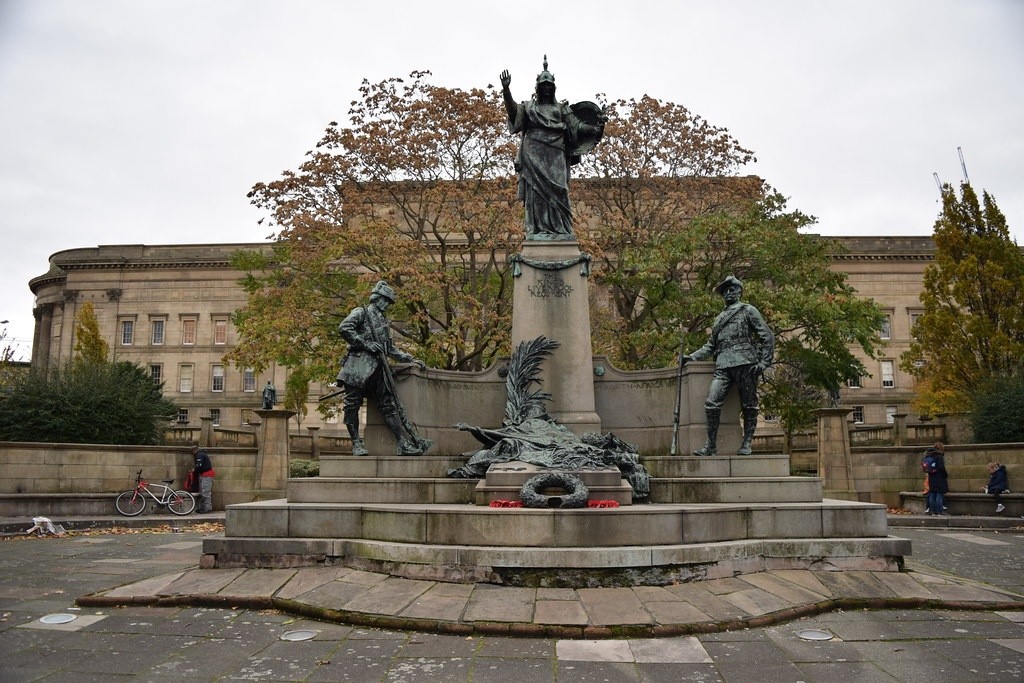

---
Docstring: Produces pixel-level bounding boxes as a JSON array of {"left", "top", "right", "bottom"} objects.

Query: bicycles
[{"left": 114, "top": 468, "right": 196, "bottom": 517}]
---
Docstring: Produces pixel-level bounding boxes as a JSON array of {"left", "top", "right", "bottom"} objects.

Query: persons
[
  {"left": 677, "top": 276, "right": 775, "bottom": 456},
  {"left": 499, "top": 54, "right": 604, "bottom": 234},
  {"left": 192, "top": 445, "right": 216, "bottom": 513},
  {"left": 924, "top": 442, "right": 951, "bottom": 517},
  {"left": 981, "top": 462, "right": 1010, "bottom": 512},
  {"left": 336, "top": 281, "right": 428, "bottom": 456},
  {"left": 262, "top": 379, "right": 277, "bottom": 409}
]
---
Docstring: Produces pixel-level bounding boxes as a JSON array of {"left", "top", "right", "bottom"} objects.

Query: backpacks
[
  {"left": 921, "top": 457, "right": 937, "bottom": 473},
  {"left": 184, "top": 471, "right": 200, "bottom": 493}
]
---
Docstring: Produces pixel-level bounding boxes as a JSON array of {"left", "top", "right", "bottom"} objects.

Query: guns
[
  {"left": 361, "top": 302, "right": 432, "bottom": 451},
  {"left": 670, "top": 336, "right": 684, "bottom": 454}
]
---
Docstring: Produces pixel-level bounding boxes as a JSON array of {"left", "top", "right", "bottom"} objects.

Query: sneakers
[
  {"left": 932, "top": 512, "right": 951, "bottom": 517},
  {"left": 943, "top": 506, "right": 948, "bottom": 510},
  {"left": 925, "top": 508, "right": 932, "bottom": 512},
  {"left": 1004, "top": 489, "right": 1010, "bottom": 494},
  {"left": 996, "top": 505, "right": 1005, "bottom": 512}
]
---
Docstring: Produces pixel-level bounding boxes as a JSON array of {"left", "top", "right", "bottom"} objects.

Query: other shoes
[{"left": 196, "top": 509, "right": 210, "bottom": 513}]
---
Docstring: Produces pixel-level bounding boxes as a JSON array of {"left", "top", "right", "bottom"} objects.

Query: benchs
[
  {"left": 900, "top": 491, "right": 1024, "bottom": 516},
  {"left": 0, "top": 492, "right": 201, "bottom": 517}
]
